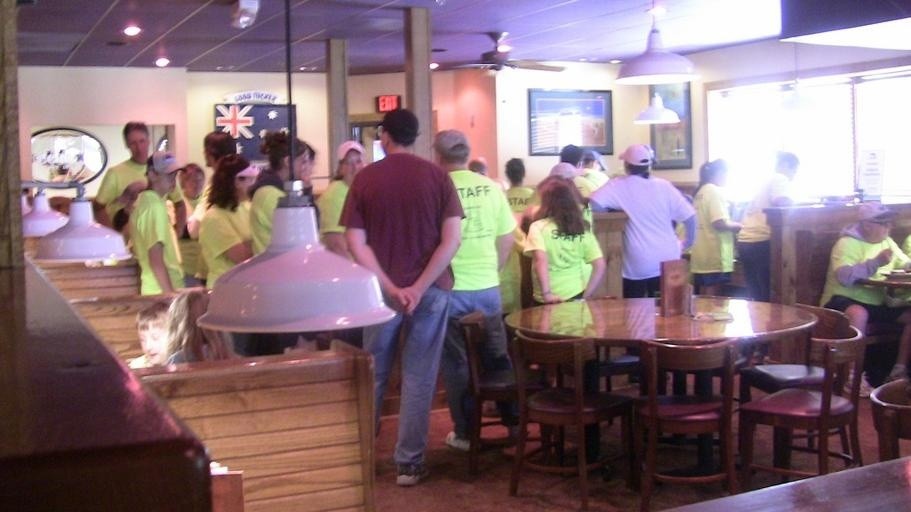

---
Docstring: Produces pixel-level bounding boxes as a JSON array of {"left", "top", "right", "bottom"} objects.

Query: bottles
[
  {"left": 690, "top": 319, "right": 699, "bottom": 337},
  {"left": 654, "top": 291, "right": 661, "bottom": 315},
  {"left": 685, "top": 283, "right": 695, "bottom": 317}
]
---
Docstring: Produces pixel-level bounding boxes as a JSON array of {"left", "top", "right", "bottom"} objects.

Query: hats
[
  {"left": 617, "top": 144, "right": 651, "bottom": 166},
  {"left": 583, "top": 150, "right": 606, "bottom": 166},
  {"left": 144, "top": 150, "right": 188, "bottom": 175},
  {"left": 234, "top": 162, "right": 261, "bottom": 179},
  {"left": 433, "top": 129, "right": 471, "bottom": 157},
  {"left": 336, "top": 139, "right": 366, "bottom": 162},
  {"left": 855, "top": 200, "right": 900, "bottom": 223},
  {"left": 643, "top": 144, "right": 656, "bottom": 161}
]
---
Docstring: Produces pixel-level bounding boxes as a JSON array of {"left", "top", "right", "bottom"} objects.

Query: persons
[
  {"left": 892, "top": 235, "right": 911, "bottom": 306},
  {"left": 738, "top": 153, "right": 801, "bottom": 363},
  {"left": 822, "top": 200, "right": 911, "bottom": 395},
  {"left": 690, "top": 158, "right": 743, "bottom": 296},
  {"left": 340, "top": 109, "right": 467, "bottom": 486}
]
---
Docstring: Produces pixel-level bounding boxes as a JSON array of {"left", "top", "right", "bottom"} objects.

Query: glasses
[{"left": 867, "top": 219, "right": 891, "bottom": 227}]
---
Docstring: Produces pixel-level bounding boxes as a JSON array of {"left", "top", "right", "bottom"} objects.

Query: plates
[{"left": 881, "top": 271, "right": 911, "bottom": 279}]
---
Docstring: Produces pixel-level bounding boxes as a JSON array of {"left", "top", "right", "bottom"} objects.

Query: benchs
[{"left": 36, "top": 266, "right": 376, "bottom": 512}]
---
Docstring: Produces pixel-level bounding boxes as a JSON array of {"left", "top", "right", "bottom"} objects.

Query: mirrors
[{"left": 26, "top": 127, "right": 111, "bottom": 188}]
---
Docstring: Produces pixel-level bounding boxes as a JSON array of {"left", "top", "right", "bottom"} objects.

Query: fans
[{"left": 432, "top": 28, "right": 570, "bottom": 78}]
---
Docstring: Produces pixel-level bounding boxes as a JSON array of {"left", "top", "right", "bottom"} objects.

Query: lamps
[
  {"left": 192, "top": 0, "right": 398, "bottom": 337},
  {"left": 13, "top": 178, "right": 131, "bottom": 269},
  {"left": 613, "top": 0, "right": 694, "bottom": 81},
  {"left": 633, "top": 82, "right": 684, "bottom": 129},
  {"left": 12, "top": 182, "right": 69, "bottom": 237}
]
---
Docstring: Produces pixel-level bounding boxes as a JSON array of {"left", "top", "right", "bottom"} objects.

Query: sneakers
[
  {"left": 883, "top": 373, "right": 907, "bottom": 383},
  {"left": 395, "top": 457, "right": 439, "bottom": 488},
  {"left": 507, "top": 424, "right": 521, "bottom": 440},
  {"left": 444, "top": 428, "right": 473, "bottom": 452},
  {"left": 842, "top": 371, "right": 878, "bottom": 398}
]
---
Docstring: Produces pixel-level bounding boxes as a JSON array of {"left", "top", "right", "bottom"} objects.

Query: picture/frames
[
  {"left": 643, "top": 80, "right": 695, "bottom": 171},
  {"left": 524, "top": 84, "right": 617, "bottom": 156}
]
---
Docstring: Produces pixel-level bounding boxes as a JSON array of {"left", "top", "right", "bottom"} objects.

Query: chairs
[{"left": 444, "top": 200, "right": 911, "bottom": 512}]
